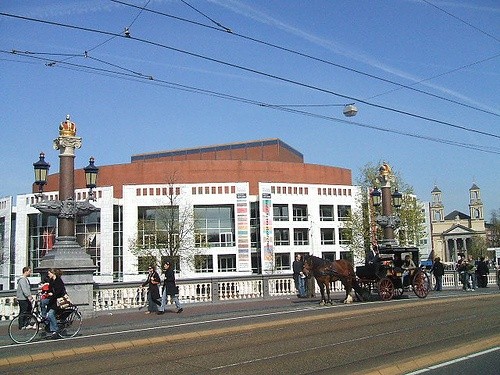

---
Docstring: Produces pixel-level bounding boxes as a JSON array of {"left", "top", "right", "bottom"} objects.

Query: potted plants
[{"left": 470, "top": 233, "right": 490, "bottom": 288}]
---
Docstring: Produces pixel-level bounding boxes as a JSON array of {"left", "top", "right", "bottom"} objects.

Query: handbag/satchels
[
  {"left": 150, "top": 272, "right": 160, "bottom": 284},
  {"left": 56, "top": 293, "right": 74, "bottom": 310}
]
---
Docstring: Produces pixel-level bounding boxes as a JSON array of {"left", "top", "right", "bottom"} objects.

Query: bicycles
[{"left": 8, "top": 292, "right": 83, "bottom": 344}]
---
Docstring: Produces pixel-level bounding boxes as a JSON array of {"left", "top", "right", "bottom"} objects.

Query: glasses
[{"left": 148, "top": 268, "right": 152, "bottom": 269}]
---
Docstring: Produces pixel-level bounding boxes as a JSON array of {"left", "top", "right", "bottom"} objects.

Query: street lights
[
  {"left": 371, "top": 185, "right": 404, "bottom": 245},
  {"left": 31, "top": 151, "right": 100, "bottom": 241}
]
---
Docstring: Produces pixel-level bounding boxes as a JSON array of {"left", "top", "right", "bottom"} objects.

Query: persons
[
  {"left": 401, "top": 255, "right": 417, "bottom": 292},
  {"left": 36, "top": 276, "right": 53, "bottom": 332},
  {"left": 302, "top": 259, "right": 317, "bottom": 299},
  {"left": 139, "top": 265, "right": 166, "bottom": 313},
  {"left": 158, "top": 260, "right": 183, "bottom": 315},
  {"left": 37, "top": 268, "right": 68, "bottom": 339},
  {"left": 455, "top": 254, "right": 489, "bottom": 292},
  {"left": 293, "top": 253, "right": 307, "bottom": 299},
  {"left": 17, "top": 266, "right": 34, "bottom": 331},
  {"left": 390, "top": 254, "right": 403, "bottom": 277},
  {"left": 430, "top": 257, "right": 444, "bottom": 291}
]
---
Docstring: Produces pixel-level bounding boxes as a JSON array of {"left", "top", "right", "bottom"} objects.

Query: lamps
[{"left": 344, "top": 103, "right": 358, "bottom": 116}]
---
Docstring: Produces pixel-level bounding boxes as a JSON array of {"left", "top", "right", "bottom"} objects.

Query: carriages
[{"left": 301, "top": 246, "right": 432, "bottom": 306}]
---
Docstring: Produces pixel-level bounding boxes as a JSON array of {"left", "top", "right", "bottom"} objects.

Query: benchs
[{"left": 405, "top": 268, "right": 418, "bottom": 283}]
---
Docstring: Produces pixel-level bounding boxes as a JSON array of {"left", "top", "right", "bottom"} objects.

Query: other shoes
[
  {"left": 158, "top": 311, "right": 164, "bottom": 315},
  {"left": 46, "top": 333, "right": 59, "bottom": 340},
  {"left": 19, "top": 325, "right": 33, "bottom": 330},
  {"left": 145, "top": 311, "right": 151, "bottom": 315},
  {"left": 177, "top": 308, "right": 183, "bottom": 313},
  {"left": 301, "top": 295, "right": 307, "bottom": 298}
]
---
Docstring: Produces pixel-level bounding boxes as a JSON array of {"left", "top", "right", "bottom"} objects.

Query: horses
[{"left": 302, "top": 255, "right": 363, "bottom": 306}]
